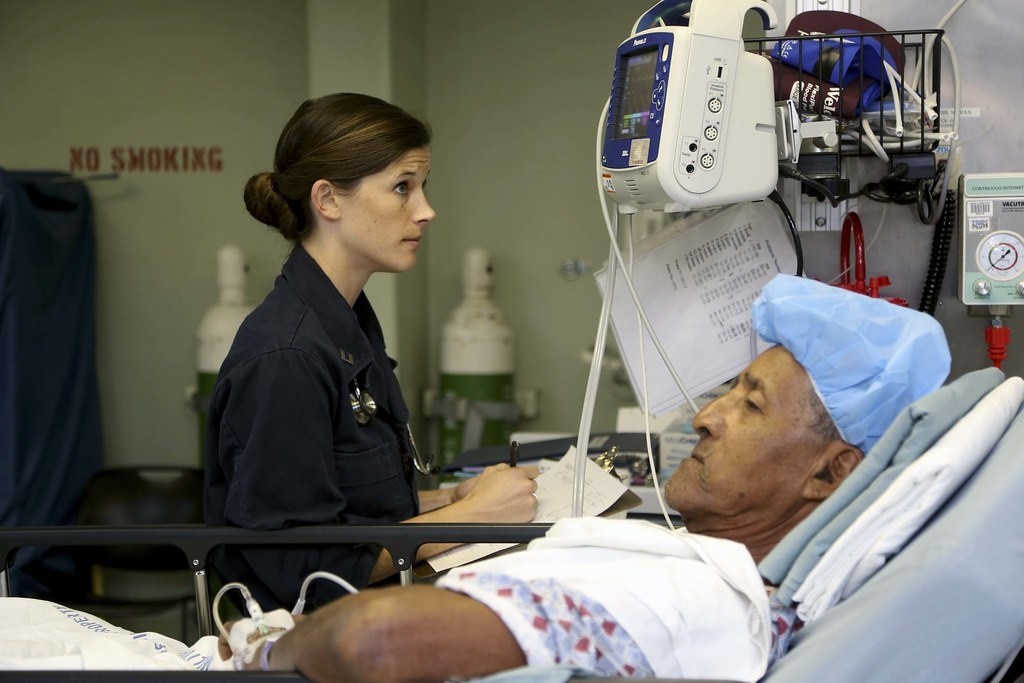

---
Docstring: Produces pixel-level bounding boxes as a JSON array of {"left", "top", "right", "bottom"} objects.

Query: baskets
[{"left": 743, "top": 28, "right": 944, "bottom": 156}]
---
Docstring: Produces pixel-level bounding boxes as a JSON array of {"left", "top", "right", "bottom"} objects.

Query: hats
[{"left": 753, "top": 272, "right": 950, "bottom": 457}]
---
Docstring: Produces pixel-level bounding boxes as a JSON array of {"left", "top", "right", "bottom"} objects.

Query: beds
[{"left": 0, "top": 366, "right": 1024, "bottom": 683}]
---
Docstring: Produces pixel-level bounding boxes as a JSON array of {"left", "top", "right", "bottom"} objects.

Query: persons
[
  {"left": 202, "top": 92, "right": 539, "bottom": 634},
  {"left": 0, "top": 274, "right": 951, "bottom": 683}
]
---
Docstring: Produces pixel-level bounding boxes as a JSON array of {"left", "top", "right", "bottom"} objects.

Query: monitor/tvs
[{"left": 613, "top": 48, "right": 658, "bottom": 139}]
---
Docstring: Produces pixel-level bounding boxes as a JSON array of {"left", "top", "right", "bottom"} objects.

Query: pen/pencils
[{"left": 509, "top": 441, "right": 518, "bottom": 468}]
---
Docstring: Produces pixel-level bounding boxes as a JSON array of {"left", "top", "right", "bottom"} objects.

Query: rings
[{"left": 532, "top": 493, "right": 538, "bottom": 508}]
